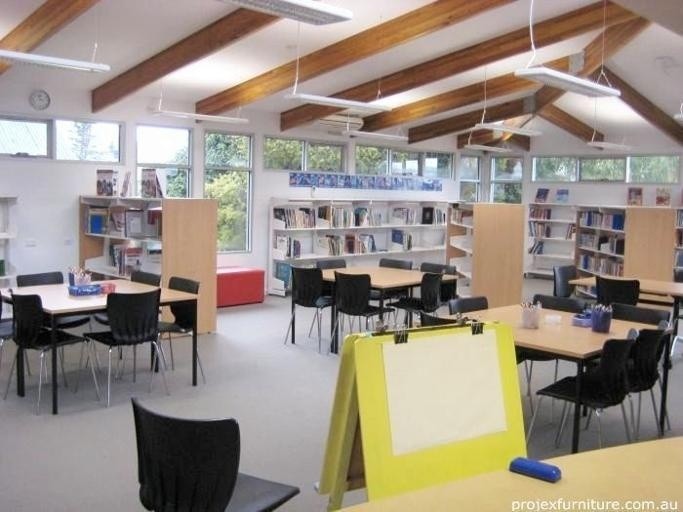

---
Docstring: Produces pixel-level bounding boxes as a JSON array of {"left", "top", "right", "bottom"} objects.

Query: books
[
  {"left": 527, "top": 188, "right": 575, "bottom": 258},
  {"left": 450, "top": 206, "right": 466, "bottom": 223},
  {"left": 273, "top": 206, "right": 383, "bottom": 257},
  {"left": 84, "top": 205, "right": 163, "bottom": 278},
  {"left": 578, "top": 210, "right": 625, "bottom": 278},
  {"left": 275, "top": 262, "right": 294, "bottom": 290},
  {"left": 388, "top": 206, "right": 446, "bottom": 255}
]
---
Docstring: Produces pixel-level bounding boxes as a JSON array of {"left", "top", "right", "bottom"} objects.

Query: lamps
[{"left": 0, "top": 0, "right": 640, "bottom": 157}]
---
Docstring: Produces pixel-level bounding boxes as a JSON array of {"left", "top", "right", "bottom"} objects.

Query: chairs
[
  {"left": 1, "top": 260, "right": 225, "bottom": 415},
  {"left": 129, "top": 395, "right": 302, "bottom": 512},
  {"left": 279, "top": 255, "right": 683, "bottom": 456}
]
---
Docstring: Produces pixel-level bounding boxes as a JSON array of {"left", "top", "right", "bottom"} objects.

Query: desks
[{"left": 325, "top": 430, "right": 683, "bottom": 510}]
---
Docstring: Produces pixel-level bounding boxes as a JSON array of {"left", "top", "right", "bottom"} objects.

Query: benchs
[{"left": 217, "top": 265, "right": 262, "bottom": 309}]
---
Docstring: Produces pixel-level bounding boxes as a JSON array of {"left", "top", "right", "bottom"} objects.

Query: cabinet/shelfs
[
  {"left": 264, "top": 199, "right": 682, "bottom": 314},
  {"left": 76, "top": 194, "right": 219, "bottom": 341},
  {"left": 1, "top": 191, "right": 21, "bottom": 340}
]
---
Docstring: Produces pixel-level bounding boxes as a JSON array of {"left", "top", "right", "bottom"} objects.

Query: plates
[
  {"left": 68, "top": 285, "right": 100, "bottom": 295},
  {"left": 570, "top": 315, "right": 591, "bottom": 327}
]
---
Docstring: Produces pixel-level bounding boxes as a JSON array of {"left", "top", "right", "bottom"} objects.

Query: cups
[
  {"left": 591, "top": 310, "right": 611, "bottom": 333},
  {"left": 521, "top": 309, "right": 538, "bottom": 328},
  {"left": 100, "top": 283, "right": 114, "bottom": 295}
]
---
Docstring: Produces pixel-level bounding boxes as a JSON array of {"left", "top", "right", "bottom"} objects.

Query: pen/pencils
[
  {"left": 591, "top": 304, "right": 613, "bottom": 331},
  {"left": 520, "top": 300, "right": 542, "bottom": 329}
]
